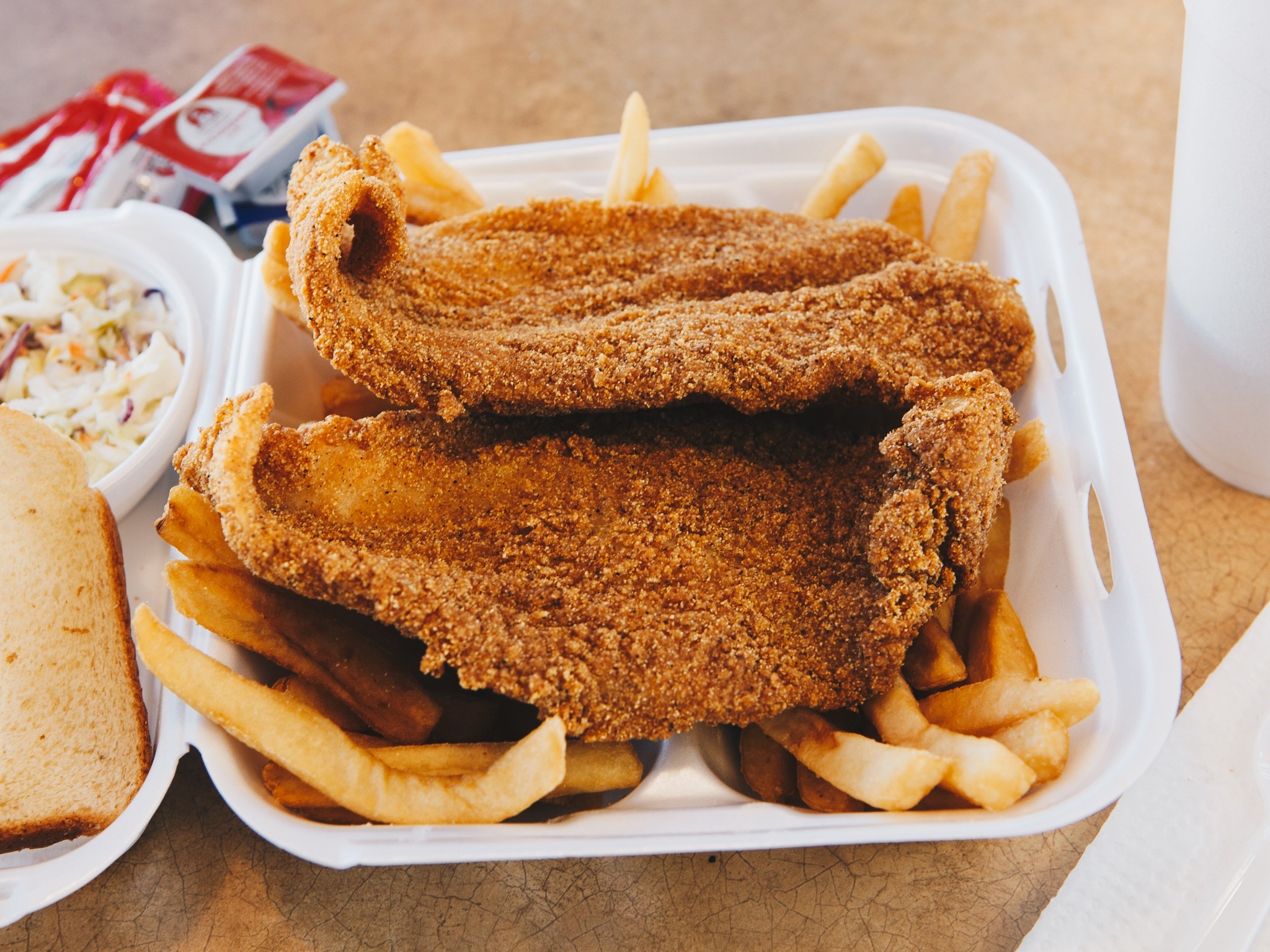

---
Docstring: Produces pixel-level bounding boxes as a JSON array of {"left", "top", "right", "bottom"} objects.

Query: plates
[{"left": 1, "top": 104, "right": 1181, "bottom": 930}]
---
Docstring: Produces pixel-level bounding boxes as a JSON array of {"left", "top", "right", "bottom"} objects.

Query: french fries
[{"left": 129, "top": 84, "right": 1101, "bottom": 825}]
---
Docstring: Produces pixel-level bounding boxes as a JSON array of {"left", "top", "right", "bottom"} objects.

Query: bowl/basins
[{"left": 0, "top": 249, "right": 205, "bottom": 528}]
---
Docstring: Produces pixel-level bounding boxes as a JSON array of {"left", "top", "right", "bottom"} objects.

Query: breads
[{"left": 0, "top": 401, "right": 154, "bottom": 853}]
